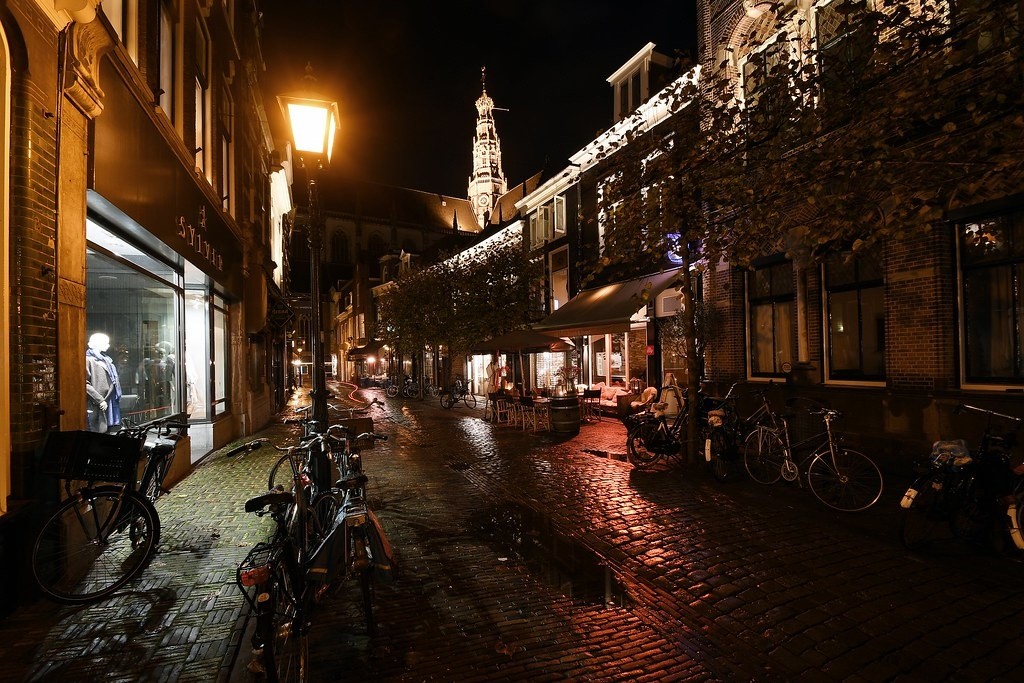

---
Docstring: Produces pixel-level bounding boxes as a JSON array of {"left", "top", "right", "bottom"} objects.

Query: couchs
[{"left": 579, "top": 383, "right": 634, "bottom": 422}]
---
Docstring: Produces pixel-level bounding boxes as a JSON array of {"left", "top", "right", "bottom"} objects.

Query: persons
[
  {"left": 425, "top": 375, "right": 430, "bottom": 384},
  {"left": 86, "top": 333, "right": 122, "bottom": 434},
  {"left": 135, "top": 363, "right": 148, "bottom": 401}
]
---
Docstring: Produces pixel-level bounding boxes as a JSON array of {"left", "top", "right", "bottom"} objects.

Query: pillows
[
  {"left": 612, "top": 389, "right": 628, "bottom": 402},
  {"left": 592, "top": 382, "right": 605, "bottom": 392},
  {"left": 601, "top": 386, "right": 617, "bottom": 399}
]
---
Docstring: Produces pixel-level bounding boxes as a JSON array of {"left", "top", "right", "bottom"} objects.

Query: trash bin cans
[{"left": 360, "top": 378, "right": 366, "bottom": 388}]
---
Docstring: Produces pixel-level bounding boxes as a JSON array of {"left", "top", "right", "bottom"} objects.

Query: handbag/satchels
[{"left": 305, "top": 509, "right": 398, "bottom": 586}]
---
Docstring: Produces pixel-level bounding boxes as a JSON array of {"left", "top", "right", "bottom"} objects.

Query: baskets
[
  {"left": 330, "top": 418, "right": 375, "bottom": 453},
  {"left": 41, "top": 431, "right": 142, "bottom": 483}
]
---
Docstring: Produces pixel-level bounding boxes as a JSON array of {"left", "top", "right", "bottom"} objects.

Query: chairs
[
  {"left": 631, "top": 387, "right": 657, "bottom": 414},
  {"left": 487, "top": 387, "right": 602, "bottom": 432}
]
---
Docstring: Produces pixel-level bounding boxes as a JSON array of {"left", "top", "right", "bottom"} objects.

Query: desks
[{"left": 511, "top": 396, "right": 551, "bottom": 432}]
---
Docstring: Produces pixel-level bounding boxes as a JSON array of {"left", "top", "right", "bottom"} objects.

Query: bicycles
[
  {"left": 25, "top": 412, "right": 191, "bottom": 605},
  {"left": 387, "top": 374, "right": 440, "bottom": 399},
  {"left": 440, "top": 377, "right": 476, "bottom": 409},
  {"left": 621, "top": 380, "right": 887, "bottom": 517},
  {"left": 900, "top": 403, "right": 1024, "bottom": 563},
  {"left": 227, "top": 398, "right": 388, "bottom": 683}
]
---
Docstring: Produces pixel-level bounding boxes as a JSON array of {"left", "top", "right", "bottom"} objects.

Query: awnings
[
  {"left": 354, "top": 340, "right": 391, "bottom": 354},
  {"left": 530, "top": 266, "right": 691, "bottom": 335}
]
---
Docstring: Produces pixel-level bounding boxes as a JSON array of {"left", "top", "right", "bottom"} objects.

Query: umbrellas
[{"left": 460, "top": 327, "right": 581, "bottom": 398}]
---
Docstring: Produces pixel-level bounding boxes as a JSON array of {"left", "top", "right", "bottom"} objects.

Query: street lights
[
  {"left": 274, "top": 62, "right": 356, "bottom": 591},
  {"left": 297, "top": 348, "right": 302, "bottom": 388}
]
